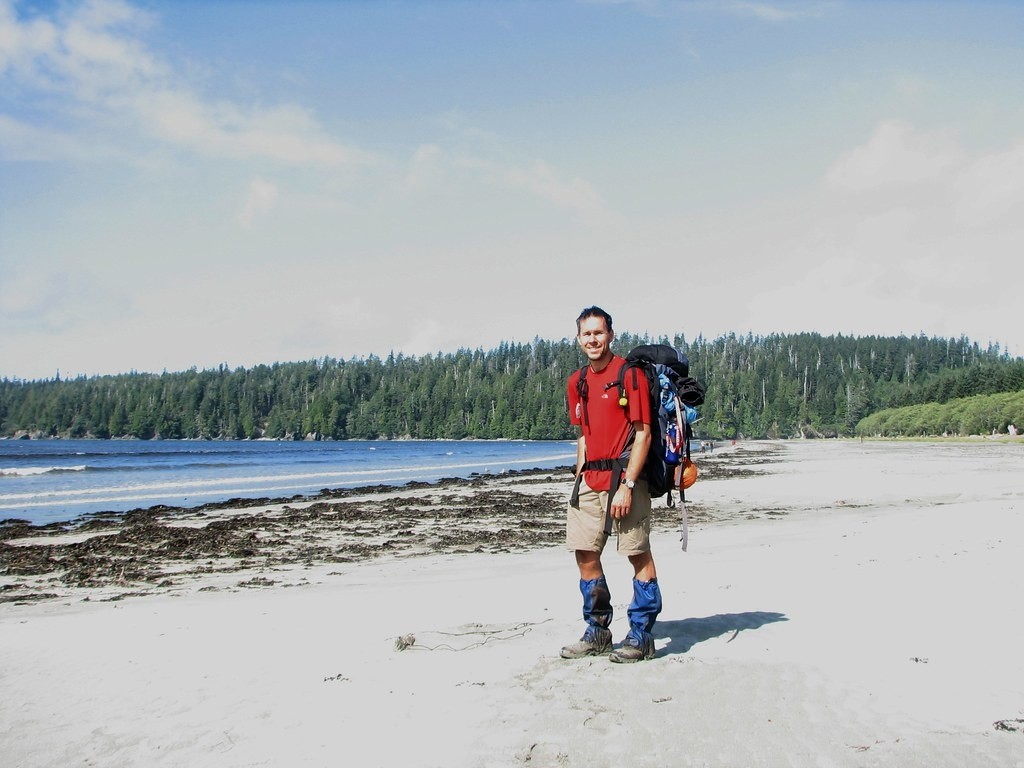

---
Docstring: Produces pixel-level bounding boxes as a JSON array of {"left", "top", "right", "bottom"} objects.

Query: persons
[
  {"left": 701, "top": 440, "right": 714, "bottom": 453},
  {"left": 559, "top": 306, "right": 662, "bottom": 664}
]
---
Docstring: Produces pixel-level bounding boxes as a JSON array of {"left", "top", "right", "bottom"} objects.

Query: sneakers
[
  {"left": 609, "top": 636, "right": 655, "bottom": 663},
  {"left": 559, "top": 627, "right": 612, "bottom": 659}
]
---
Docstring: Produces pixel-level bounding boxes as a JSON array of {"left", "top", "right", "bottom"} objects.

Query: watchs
[{"left": 621, "top": 479, "right": 634, "bottom": 489}]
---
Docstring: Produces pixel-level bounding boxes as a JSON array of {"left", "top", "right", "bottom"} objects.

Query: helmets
[{"left": 673, "top": 462, "right": 697, "bottom": 490}]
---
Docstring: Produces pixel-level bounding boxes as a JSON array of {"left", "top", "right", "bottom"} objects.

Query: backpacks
[{"left": 579, "top": 344, "right": 704, "bottom": 498}]
